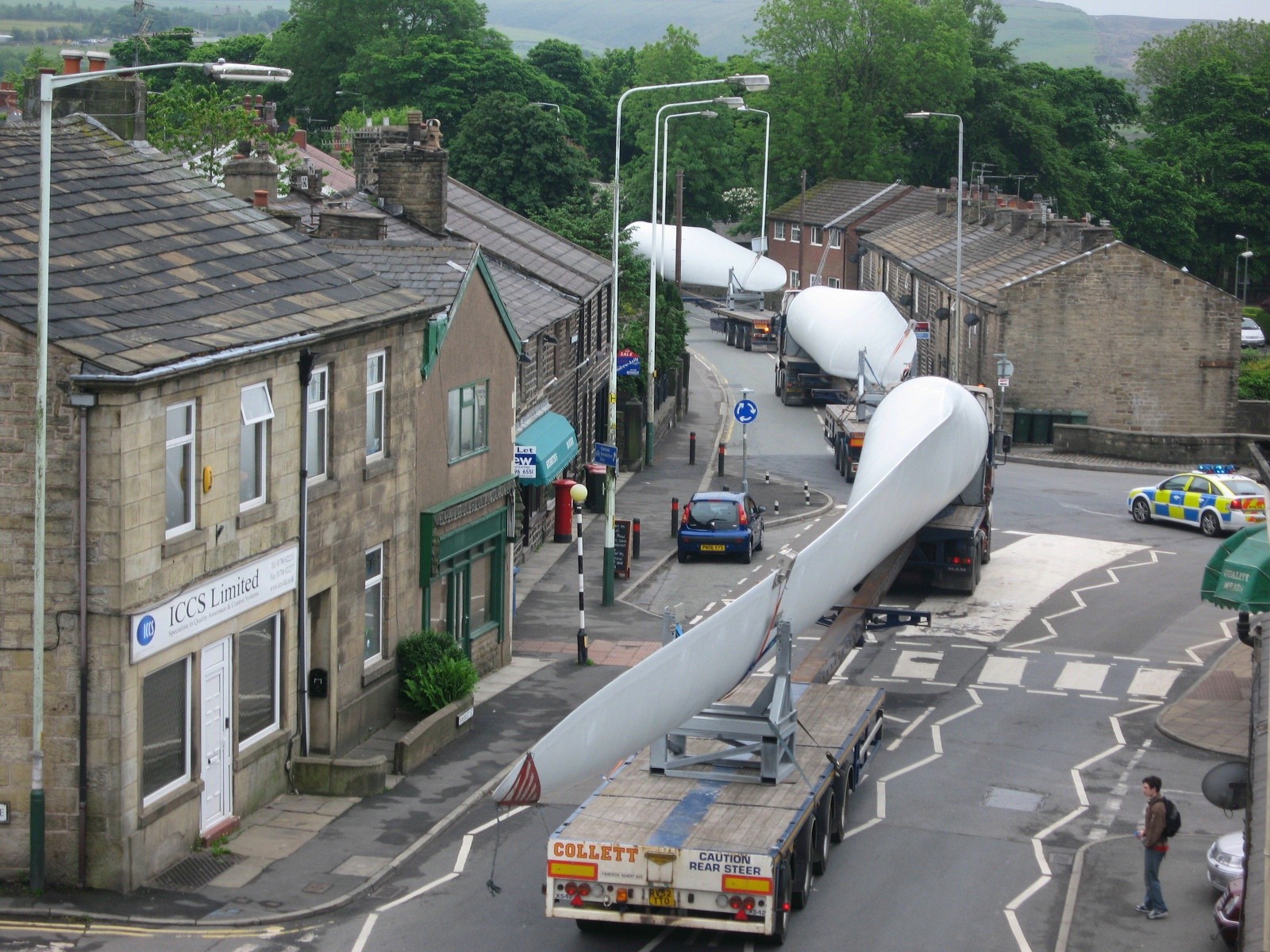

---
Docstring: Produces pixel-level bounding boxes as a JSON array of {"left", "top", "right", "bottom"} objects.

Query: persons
[{"left": 1132, "top": 776, "right": 1172, "bottom": 920}]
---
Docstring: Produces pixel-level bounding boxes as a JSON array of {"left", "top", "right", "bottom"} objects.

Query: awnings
[{"left": 515, "top": 409, "right": 579, "bottom": 486}]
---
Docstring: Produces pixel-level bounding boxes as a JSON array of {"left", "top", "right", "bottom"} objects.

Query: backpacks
[{"left": 1150, "top": 795, "right": 1181, "bottom": 837}]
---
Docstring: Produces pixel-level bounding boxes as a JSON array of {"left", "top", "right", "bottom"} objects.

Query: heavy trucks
[
  {"left": 770, "top": 285, "right": 918, "bottom": 483},
  {"left": 604, "top": 221, "right": 788, "bottom": 353},
  {"left": 488, "top": 373, "right": 1013, "bottom": 951}
]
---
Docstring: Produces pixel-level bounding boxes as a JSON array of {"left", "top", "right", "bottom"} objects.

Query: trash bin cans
[
  {"left": 1029, "top": 407, "right": 1051, "bottom": 444},
  {"left": 584, "top": 463, "right": 607, "bottom": 513},
  {"left": 1014, "top": 408, "right": 1033, "bottom": 444},
  {"left": 1049, "top": 407, "right": 1071, "bottom": 444},
  {"left": 1070, "top": 409, "right": 1088, "bottom": 425},
  {"left": 996, "top": 407, "right": 1014, "bottom": 443}
]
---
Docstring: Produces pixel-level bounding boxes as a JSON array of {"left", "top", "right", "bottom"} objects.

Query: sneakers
[
  {"left": 1146, "top": 909, "right": 1168, "bottom": 919},
  {"left": 1135, "top": 904, "right": 1152, "bottom": 913}
]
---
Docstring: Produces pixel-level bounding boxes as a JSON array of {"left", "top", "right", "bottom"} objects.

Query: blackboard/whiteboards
[{"left": 615, "top": 521, "right": 633, "bottom": 572}]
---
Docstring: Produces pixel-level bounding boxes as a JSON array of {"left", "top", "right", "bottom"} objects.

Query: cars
[
  {"left": 1206, "top": 831, "right": 1246, "bottom": 894},
  {"left": 677, "top": 490, "right": 767, "bottom": 564},
  {"left": 1240, "top": 317, "right": 1265, "bottom": 348},
  {"left": 1213, "top": 878, "right": 1244, "bottom": 943},
  {"left": 1125, "top": 463, "right": 1270, "bottom": 538}
]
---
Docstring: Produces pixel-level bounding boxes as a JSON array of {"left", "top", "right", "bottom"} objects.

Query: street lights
[
  {"left": 570, "top": 484, "right": 589, "bottom": 666},
  {"left": 903, "top": 110, "right": 964, "bottom": 380},
  {"left": 1235, "top": 234, "right": 1249, "bottom": 302},
  {"left": 26, "top": 58, "right": 295, "bottom": 905},
  {"left": 1234, "top": 251, "right": 1253, "bottom": 297},
  {"left": 600, "top": 73, "right": 771, "bottom": 608}
]
